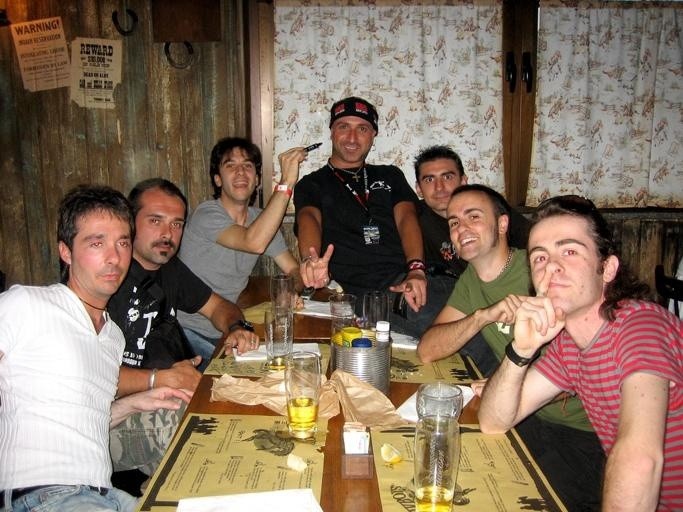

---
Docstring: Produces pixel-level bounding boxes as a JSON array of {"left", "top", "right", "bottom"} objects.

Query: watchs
[{"left": 229, "top": 320, "right": 254, "bottom": 331}]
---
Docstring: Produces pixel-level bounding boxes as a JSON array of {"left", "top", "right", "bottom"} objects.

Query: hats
[{"left": 328, "top": 96, "right": 380, "bottom": 137}]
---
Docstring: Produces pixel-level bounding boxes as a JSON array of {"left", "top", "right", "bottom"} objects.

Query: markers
[{"left": 304, "top": 143, "right": 322, "bottom": 152}]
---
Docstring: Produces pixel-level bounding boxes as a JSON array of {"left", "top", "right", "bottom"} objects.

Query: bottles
[
  {"left": 372, "top": 319, "right": 391, "bottom": 348},
  {"left": 339, "top": 325, "right": 363, "bottom": 347},
  {"left": 351, "top": 338, "right": 371, "bottom": 350}
]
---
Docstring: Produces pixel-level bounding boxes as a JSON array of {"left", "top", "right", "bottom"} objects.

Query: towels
[
  {"left": 172, "top": 488, "right": 323, "bottom": 512},
  {"left": 294, "top": 296, "right": 356, "bottom": 322},
  {"left": 383, "top": 327, "right": 417, "bottom": 351},
  {"left": 231, "top": 341, "right": 320, "bottom": 362},
  {"left": 396, "top": 375, "right": 478, "bottom": 425}
]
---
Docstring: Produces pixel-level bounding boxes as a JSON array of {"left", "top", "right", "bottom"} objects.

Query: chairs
[{"left": 651, "top": 261, "right": 682, "bottom": 318}]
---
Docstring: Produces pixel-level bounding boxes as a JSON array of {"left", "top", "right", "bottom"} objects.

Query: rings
[
  {"left": 190, "top": 358, "right": 196, "bottom": 367},
  {"left": 251, "top": 338, "right": 256, "bottom": 344}
]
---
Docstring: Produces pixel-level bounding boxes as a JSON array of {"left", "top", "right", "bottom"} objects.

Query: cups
[
  {"left": 413, "top": 416, "right": 461, "bottom": 511},
  {"left": 416, "top": 381, "right": 464, "bottom": 424},
  {"left": 268, "top": 273, "right": 294, "bottom": 312},
  {"left": 361, "top": 288, "right": 391, "bottom": 331},
  {"left": 261, "top": 304, "right": 297, "bottom": 379},
  {"left": 326, "top": 290, "right": 356, "bottom": 331},
  {"left": 281, "top": 349, "right": 320, "bottom": 442}
]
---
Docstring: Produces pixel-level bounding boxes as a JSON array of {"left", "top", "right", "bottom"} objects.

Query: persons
[
  {"left": 407, "top": 144, "right": 531, "bottom": 377},
  {"left": 293, "top": 96, "right": 429, "bottom": 333},
  {"left": 418, "top": 183, "right": 603, "bottom": 486},
  {"left": 176, "top": 136, "right": 308, "bottom": 372},
  {"left": 108, "top": 177, "right": 260, "bottom": 479},
  {"left": 1, "top": 183, "right": 197, "bottom": 511},
  {"left": 477, "top": 194, "right": 683, "bottom": 512},
  {"left": 669, "top": 257, "right": 683, "bottom": 319}
]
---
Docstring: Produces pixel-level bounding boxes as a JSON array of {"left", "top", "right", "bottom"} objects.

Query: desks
[{"left": 131, "top": 274, "right": 573, "bottom": 512}]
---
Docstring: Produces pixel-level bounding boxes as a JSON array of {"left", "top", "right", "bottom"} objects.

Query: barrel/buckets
[{"left": 330, "top": 328, "right": 394, "bottom": 397}]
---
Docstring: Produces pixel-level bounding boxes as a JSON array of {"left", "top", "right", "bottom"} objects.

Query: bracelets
[
  {"left": 302, "top": 256, "right": 312, "bottom": 262},
  {"left": 149, "top": 367, "right": 157, "bottom": 391},
  {"left": 274, "top": 184, "right": 292, "bottom": 197},
  {"left": 504, "top": 338, "right": 540, "bottom": 366},
  {"left": 407, "top": 259, "right": 426, "bottom": 271}
]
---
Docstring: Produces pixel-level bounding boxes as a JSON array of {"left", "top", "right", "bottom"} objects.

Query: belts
[{"left": 0, "top": 484, "right": 109, "bottom": 508}]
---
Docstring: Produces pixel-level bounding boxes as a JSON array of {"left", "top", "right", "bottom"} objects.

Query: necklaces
[
  {"left": 333, "top": 162, "right": 364, "bottom": 180},
  {"left": 79, "top": 300, "right": 106, "bottom": 311},
  {"left": 492, "top": 248, "right": 512, "bottom": 282}
]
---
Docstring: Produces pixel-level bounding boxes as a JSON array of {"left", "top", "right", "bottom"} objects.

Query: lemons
[{"left": 381, "top": 443, "right": 403, "bottom": 463}]
